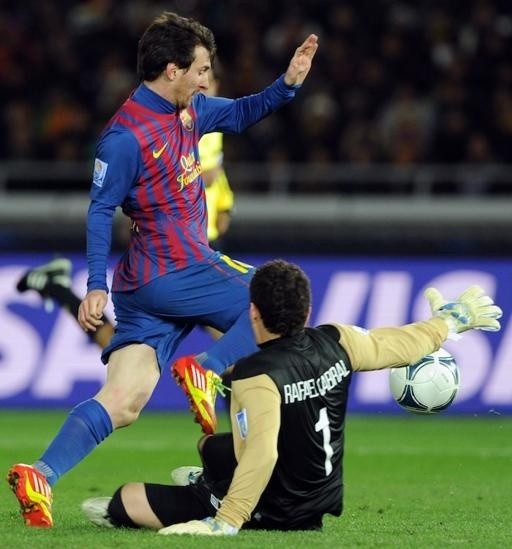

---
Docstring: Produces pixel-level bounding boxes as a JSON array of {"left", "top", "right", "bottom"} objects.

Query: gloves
[
  {"left": 423, "top": 285, "right": 503, "bottom": 344},
  {"left": 158, "top": 512, "right": 239, "bottom": 536}
]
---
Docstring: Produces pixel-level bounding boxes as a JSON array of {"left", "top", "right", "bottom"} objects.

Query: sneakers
[
  {"left": 169, "top": 352, "right": 230, "bottom": 436},
  {"left": 17, "top": 259, "right": 72, "bottom": 300},
  {"left": 80, "top": 495, "right": 114, "bottom": 529},
  {"left": 5, "top": 464, "right": 52, "bottom": 528},
  {"left": 170, "top": 465, "right": 205, "bottom": 486}
]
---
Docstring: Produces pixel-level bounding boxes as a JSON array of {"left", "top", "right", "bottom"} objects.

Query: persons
[
  {"left": 17, "top": 57, "right": 235, "bottom": 349},
  {"left": 7, "top": 13, "right": 318, "bottom": 527},
  {"left": 0, "top": 0, "right": 512, "bottom": 196},
  {"left": 80, "top": 260, "right": 502, "bottom": 534}
]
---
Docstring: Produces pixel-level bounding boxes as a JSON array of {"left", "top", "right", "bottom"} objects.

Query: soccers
[{"left": 389, "top": 349, "right": 461, "bottom": 413}]
